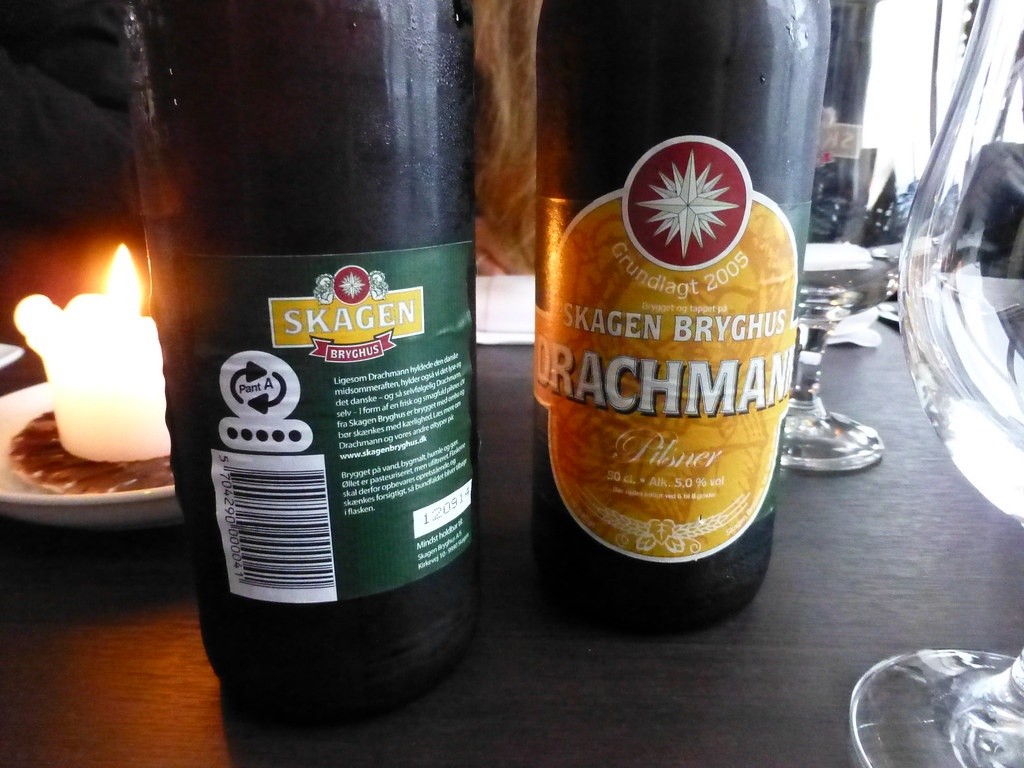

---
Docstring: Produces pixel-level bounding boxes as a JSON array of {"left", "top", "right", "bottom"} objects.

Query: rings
[{"left": 476, "top": 254, "right": 486, "bottom": 263}]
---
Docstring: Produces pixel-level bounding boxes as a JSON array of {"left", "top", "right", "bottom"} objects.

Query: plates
[{"left": 0, "top": 344, "right": 24, "bottom": 369}]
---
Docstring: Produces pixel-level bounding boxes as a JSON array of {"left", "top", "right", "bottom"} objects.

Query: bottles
[
  {"left": 122, "top": 0, "right": 477, "bottom": 725},
  {"left": 536, "top": 0, "right": 831, "bottom": 633}
]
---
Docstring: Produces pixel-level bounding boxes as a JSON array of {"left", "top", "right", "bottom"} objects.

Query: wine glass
[
  {"left": 850, "top": 0, "right": 1024, "bottom": 768},
  {"left": 780, "top": 0, "right": 980, "bottom": 474}
]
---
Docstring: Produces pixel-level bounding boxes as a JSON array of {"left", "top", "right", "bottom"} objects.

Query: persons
[{"left": 0, "top": 0, "right": 542, "bottom": 276}]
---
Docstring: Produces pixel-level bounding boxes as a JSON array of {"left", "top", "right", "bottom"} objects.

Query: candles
[{"left": 14, "top": 243, "right": 170, "bottom": 464}]
